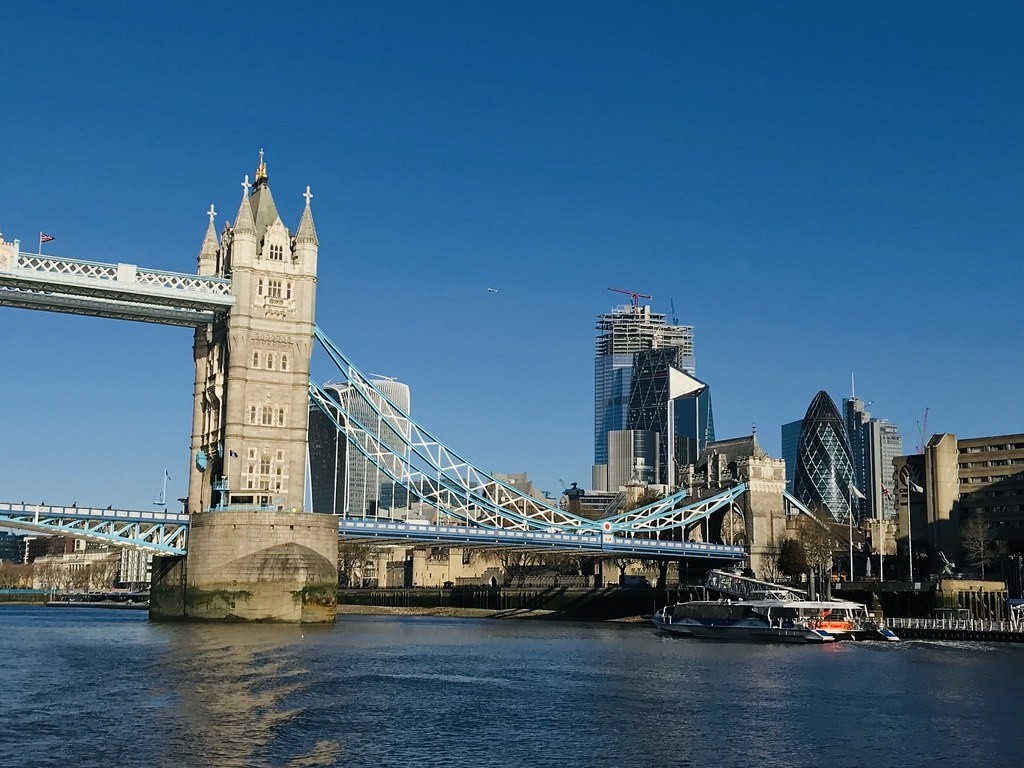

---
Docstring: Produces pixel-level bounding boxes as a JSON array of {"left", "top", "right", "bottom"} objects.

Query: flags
[
  {"left": 881, "top": 483, "right": 891, "bottom": 494},
  {"left": 910, "top": 480, "right": 925, "bottom": 493},
  {"left": 165, "top": 469, "right": 172, "bottom": 480},
  {"left": 849, "top": 483, "right": 867, "bottom": 500},
  {"left": 40, "top": 233, "right": 55, "bottom": 241}
]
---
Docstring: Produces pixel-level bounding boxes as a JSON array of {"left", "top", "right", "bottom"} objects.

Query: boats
[{"left": 650, "top": 588, "right": 904, "bottom": 644}]
